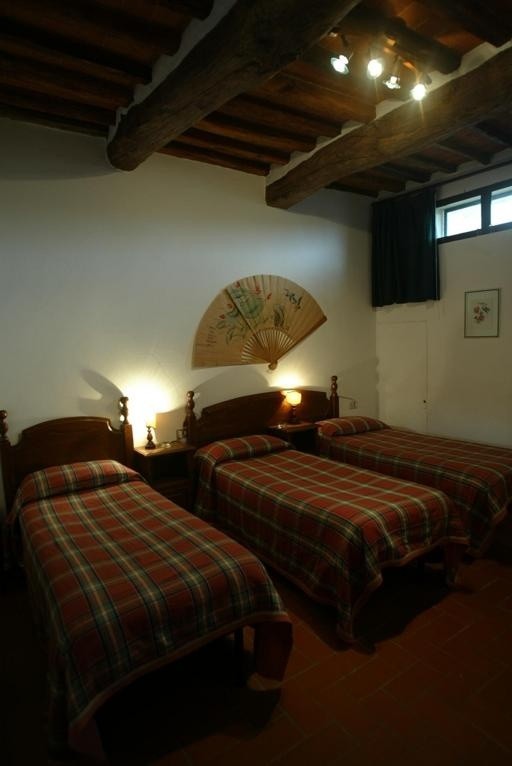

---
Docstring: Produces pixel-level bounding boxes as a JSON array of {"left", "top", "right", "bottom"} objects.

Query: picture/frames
[{"left": 464, "top": 288, "right": 499, "bottom": 338}]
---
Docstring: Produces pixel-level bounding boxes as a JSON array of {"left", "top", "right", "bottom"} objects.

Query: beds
[
  {"left": 193, "top": 434, "right": 471, "bottom": 654},
  {"left": 315, "top": 416, "right": 512, "bottom": 567},
  {"left": 0, "top": 396, "right": 293, "bottom": 766}
]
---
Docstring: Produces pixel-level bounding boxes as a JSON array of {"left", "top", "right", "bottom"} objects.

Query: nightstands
[
  {"left": 264, "top": 421, "right": 320, "bottom": 457},
  {"left": 132, "top": 440, "right": 198, "bottom": 514}
]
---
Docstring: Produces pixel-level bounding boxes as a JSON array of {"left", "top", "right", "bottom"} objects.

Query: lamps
[{"left": 330, "top": 33, "right": 432, "bottom": 102}]
